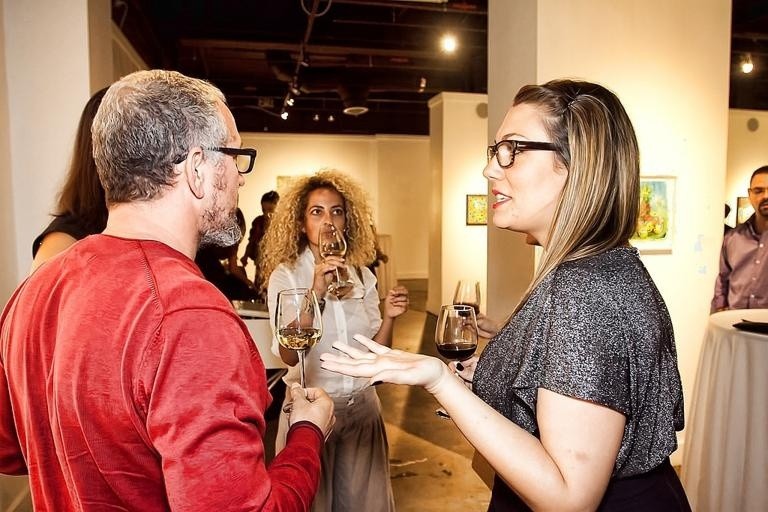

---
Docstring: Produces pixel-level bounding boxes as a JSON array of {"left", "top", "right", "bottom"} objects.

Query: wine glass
[
  {"left": 434, "top": 304, "right": 480, "bottom": 419},
  {"left": 318, "top": 224, "right": 354, "bottom": 298},
  {"left": 452, "top": 277, "right": 481, "bottom": 339},
  {"left": 274, "top": 288, "right": 323, "bottom": 414}
]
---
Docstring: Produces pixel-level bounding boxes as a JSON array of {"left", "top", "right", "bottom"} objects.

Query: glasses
[
  {"left": 486, "top": 140, "right": 562, "bottom": 168},
  {"left": 175, "top": 148, "right": 256, "bottom": 174}
]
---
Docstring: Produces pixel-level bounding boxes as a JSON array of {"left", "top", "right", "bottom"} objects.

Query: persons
[
  {"left": 714, "top": 164, "right": 768, "bottom": 314},
  {"left": 194, "top": 194, "right": 259, "bottom": 301},
  {"left": 241, "top": 189, "right": 280, "bottom": 287},
  {"left": 723, "top": 201, "right": 736, "bottom": 237},
  {"left": 461, "top": 233, "right": 543, "bottom": 338},
  {"left": 31, "top": 84, "right": 109, "bottom": 274},
  {"left": 256, "top": 168, "right": 413, "bottom": 511},
  {"left": 0, "top": 67, "right": 336, "bottom": 512},
  {"left": 318, "top": 74, "right": 695, "bottom": 512}
]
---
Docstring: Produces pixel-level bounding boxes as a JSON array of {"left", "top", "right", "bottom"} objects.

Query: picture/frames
[
  {"left": 628, "top": 176, "right": 676, "bottom": 257},
  {"left": 466, "top": 193, "right": 488, "bottom": 226}
]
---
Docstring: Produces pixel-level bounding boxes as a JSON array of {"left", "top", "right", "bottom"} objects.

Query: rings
[{"left": 320, "top": 258, "right": 326, "bottom": 265}]
